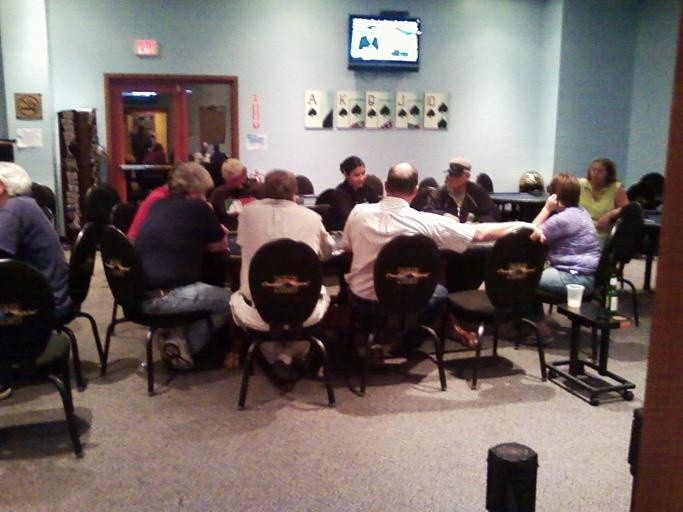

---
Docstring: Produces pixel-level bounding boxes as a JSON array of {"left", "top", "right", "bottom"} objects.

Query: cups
[{"left": 565, "top": 284, "right": 586, "bottom": 307}]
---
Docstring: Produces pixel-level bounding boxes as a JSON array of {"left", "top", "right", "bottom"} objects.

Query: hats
[{"left": 442, "top": 162, "right": 472, "bottom": 178}]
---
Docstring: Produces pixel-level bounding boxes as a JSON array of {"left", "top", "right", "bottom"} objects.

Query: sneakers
[
  {"left": 523, "top": 321, "right": 554, "bottom": 347},
  {"left": 161, "top": 336, "right": 197, "bottom": 373}
]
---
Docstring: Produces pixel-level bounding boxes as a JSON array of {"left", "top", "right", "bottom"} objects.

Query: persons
[
  {"left": 577, "top": 159, "right": 629, "bottom": 248},
  {"left": 126, "top": 124, "right": 548, "bottom": 391},
  {"left": 523, "top": 174, "right": 601, "bottom": 345},
  {"left": 0, "top": 162, "right": 70, "bottom": 398}
]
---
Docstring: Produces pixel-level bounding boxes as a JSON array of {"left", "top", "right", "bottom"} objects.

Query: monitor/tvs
[{"left": 348, "top": 14, "right": 420, "bottom": 72}]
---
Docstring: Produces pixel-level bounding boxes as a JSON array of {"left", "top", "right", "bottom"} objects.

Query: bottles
[{"left": 603, "top": 277, "right": 619, "bottom": 316}]
